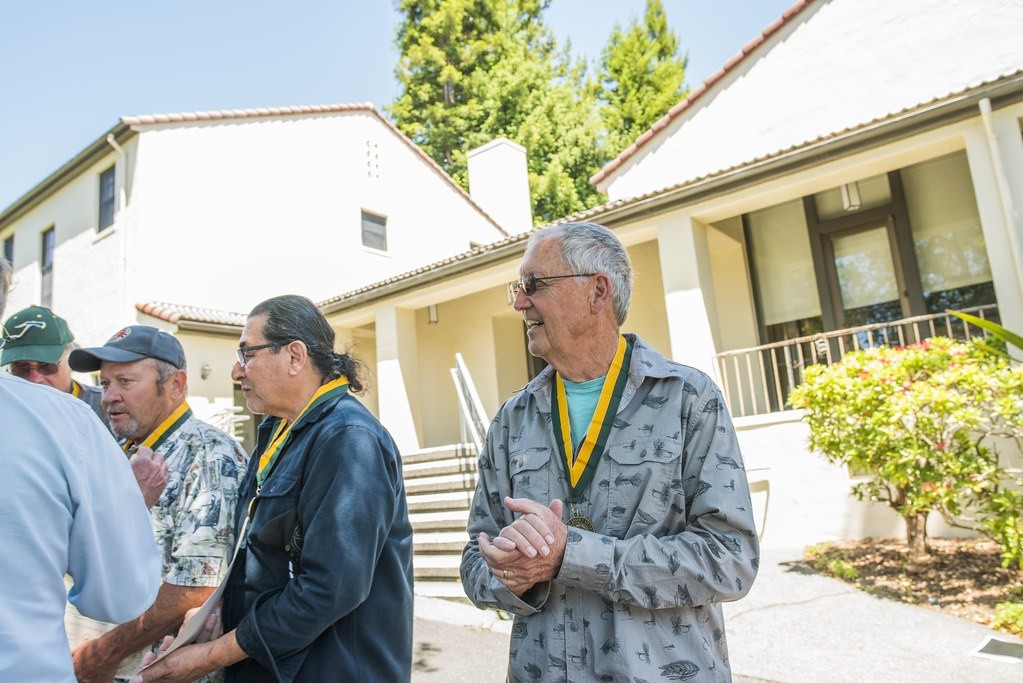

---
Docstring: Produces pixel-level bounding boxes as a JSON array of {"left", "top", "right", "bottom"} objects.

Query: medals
[
  {"left": 248, "top": 497, "right": 256, "bottom": 516},
  {"left": 565, "top": 517, "right": 595, "bottom": 533}
]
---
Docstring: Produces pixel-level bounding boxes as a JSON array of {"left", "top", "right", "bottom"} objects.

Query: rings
[{"left": 504, "top": 570, "right": 507, "bottom": 579}]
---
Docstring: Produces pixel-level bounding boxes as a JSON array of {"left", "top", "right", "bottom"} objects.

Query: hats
[
  {"left": 69, "top": 325, "right": 185, "bottom": 372},
  {"left": 0, "top": 303, "right": 74, "bottom": 366}
]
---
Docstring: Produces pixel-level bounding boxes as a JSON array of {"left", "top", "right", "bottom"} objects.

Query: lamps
[
  {"left": 839, "top": 181, "right": 862, "bottom": 212},
  {"left": 506, "top": 281, "right": 517, "bottom": 305},
  {"left": 202, "top": 364, "right": 213, "bottom": 379},
  {"left": 426, "top": 303, "right": 439, "bottom": 326}
]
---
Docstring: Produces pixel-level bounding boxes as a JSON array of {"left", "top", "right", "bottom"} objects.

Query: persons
[
  {"left": 0, "top": 305, "right": 124, "bottom": 443},
  {"left": 0, "top": 256, "right": 162, "bottom": 683},
  {"left": 67, "top": 325, "right": 251, "bottom": 683},
  {"left": 126, "top": 295, "right": 414, "bottom": 683},
  {"left": 459, "top": 223, "right": 760, "bottom": 683}
]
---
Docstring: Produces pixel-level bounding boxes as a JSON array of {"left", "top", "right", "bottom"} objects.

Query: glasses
[
  {"left": 0, "top": 337, "right": 6, "bottom": 349},
  {"left": 508, "top": 273, "right": 598, "bottom": 305},
  {"left": 5, "top": 353, "right": 68, "bottom": 379},
  {"left": 236, "top": 339, "right": 310, "bottom": 368}
]
